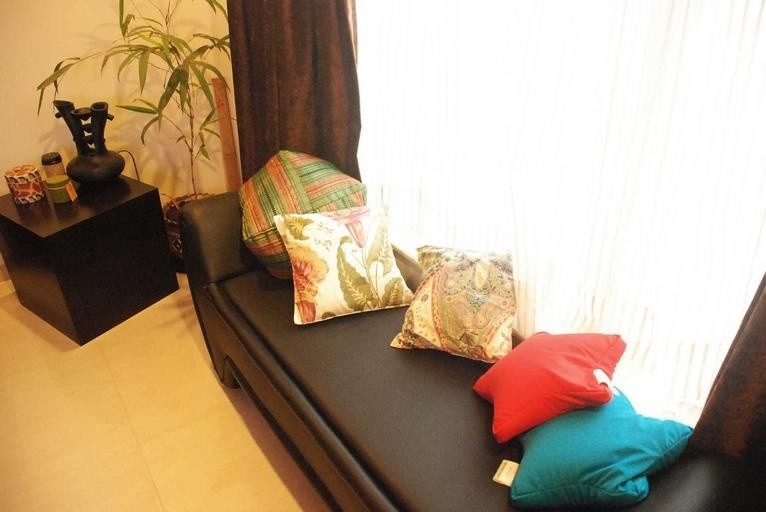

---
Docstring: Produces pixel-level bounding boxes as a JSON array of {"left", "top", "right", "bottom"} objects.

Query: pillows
[
  {"left": 388, "top": 242, "right": 516, "bottom": 364},
  {"left": 472, "top": 330, "right": 627, "bottom": 444},
  {"left": 272, "top": 202, "right": 416, "bottom": 327},
  {"left": 238, "top": 151, "right": 366, "bottom": 279},
  {"left": 509, "top": 384, "right": 697, "bottom": 508}
]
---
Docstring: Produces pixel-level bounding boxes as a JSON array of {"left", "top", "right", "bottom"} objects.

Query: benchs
[{"left": 180, "top": 187, "right": 766, "bottom": 511}]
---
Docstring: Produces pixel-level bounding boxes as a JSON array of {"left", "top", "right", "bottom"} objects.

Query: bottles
[{"left": 3, "top": 151, "right": 74, "bottom": 206}]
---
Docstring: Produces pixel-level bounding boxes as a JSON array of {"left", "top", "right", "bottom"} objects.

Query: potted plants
[{"left": 37, "top": 1, "right": 239, "bottom": 273}]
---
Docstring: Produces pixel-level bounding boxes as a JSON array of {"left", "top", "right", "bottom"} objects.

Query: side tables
[{"left": 2, "top": 172, "right": 180, "bottom": 347}]
